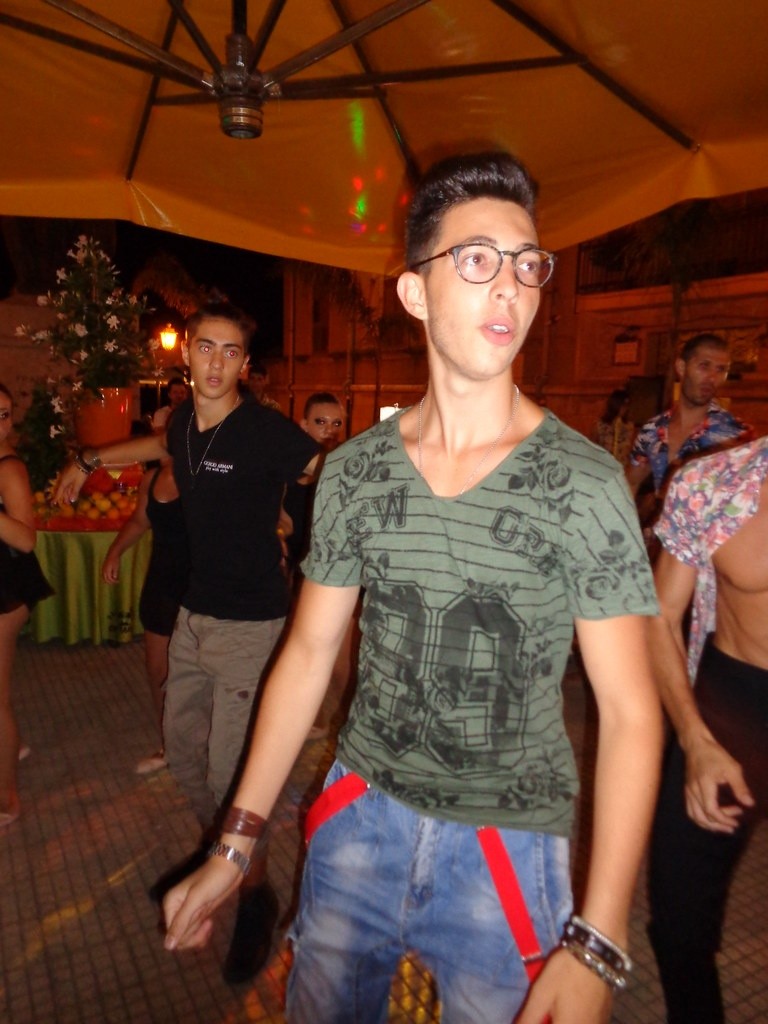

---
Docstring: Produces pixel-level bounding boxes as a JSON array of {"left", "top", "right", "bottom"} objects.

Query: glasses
[{"left": 409, "top": 243, "right": 555, "bottom": 289}]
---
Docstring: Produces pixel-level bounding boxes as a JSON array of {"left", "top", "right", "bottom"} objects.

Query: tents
[{"left": 0, "top": 0, "right": 768, "bottom": 280}]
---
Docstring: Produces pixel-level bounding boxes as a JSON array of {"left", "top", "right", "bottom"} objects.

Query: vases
[{"left": 58, "top": 385, "right": 132, "bottom": 450}]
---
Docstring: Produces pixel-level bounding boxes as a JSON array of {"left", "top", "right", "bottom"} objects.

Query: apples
[{"left": 34, "top": 489, "right": 137, "bottom": 520}]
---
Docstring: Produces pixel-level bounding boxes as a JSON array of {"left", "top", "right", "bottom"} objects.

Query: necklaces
[
  {"left": 187, "top": 395, "right": 239, "bottom": 477},
  {"left": 418, "top": 384, "right": 519, "bottom": 494}
]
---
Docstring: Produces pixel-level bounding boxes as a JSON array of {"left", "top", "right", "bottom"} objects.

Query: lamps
[{"left": 159, "top": 321, "right": 179, "bottom": 351}]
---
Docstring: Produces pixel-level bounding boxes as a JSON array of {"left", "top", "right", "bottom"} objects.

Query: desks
[{"left": 30, "top": 516, "right": 154, "bottom": 647}]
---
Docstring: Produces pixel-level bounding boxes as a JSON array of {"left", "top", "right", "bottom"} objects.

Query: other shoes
[
  {"left": 148, "top": 827, "right": 218, "bottom": 903},
  {"left": 225, "top": 882, "right": 280, "bottom": 983},
  {"left": 134, "top": 753, "right": 166, "bottom": 773}
]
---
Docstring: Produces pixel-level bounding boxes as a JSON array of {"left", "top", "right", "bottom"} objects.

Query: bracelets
[
  {"left": 71, "top": 452, "right": 96, "bottom": 476},
  {"left": 207, "top": 841, "right": 253, "bottom": 878},
  {"left": 87, "top": 448, "right": 101, "bottom": 468},
  {"left": 561, "top": 940, "right": 626, "bottom": 997},
  {"left": 222, "top": 805, "right": 267, "bottom": 839},
  {"left": 562, "top": 921, "right": 623, "bottom": 974},
  {"left": 572, "top": 916, "right": 633, "bottom": 972}
]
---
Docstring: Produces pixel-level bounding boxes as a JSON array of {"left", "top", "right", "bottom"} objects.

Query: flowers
[{"left": 13, "top": 233, "right": 165, "bottom": 442}]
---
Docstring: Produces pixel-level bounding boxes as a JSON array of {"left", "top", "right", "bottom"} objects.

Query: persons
[
  {"left": 51, "top": 155, "right": 662, "bottom": 1024},
  {"left": 628, "top": 332, "right": 751, "bottom": 569},
  {"left": 644, "top": 441, "right": 768, "bottom": 1024},
  {"left": 0, "top": 386, "right": 55, "bottom": 829},
  {"left": 590, "top": 389, "right": 636, "bottom": 464}
]
